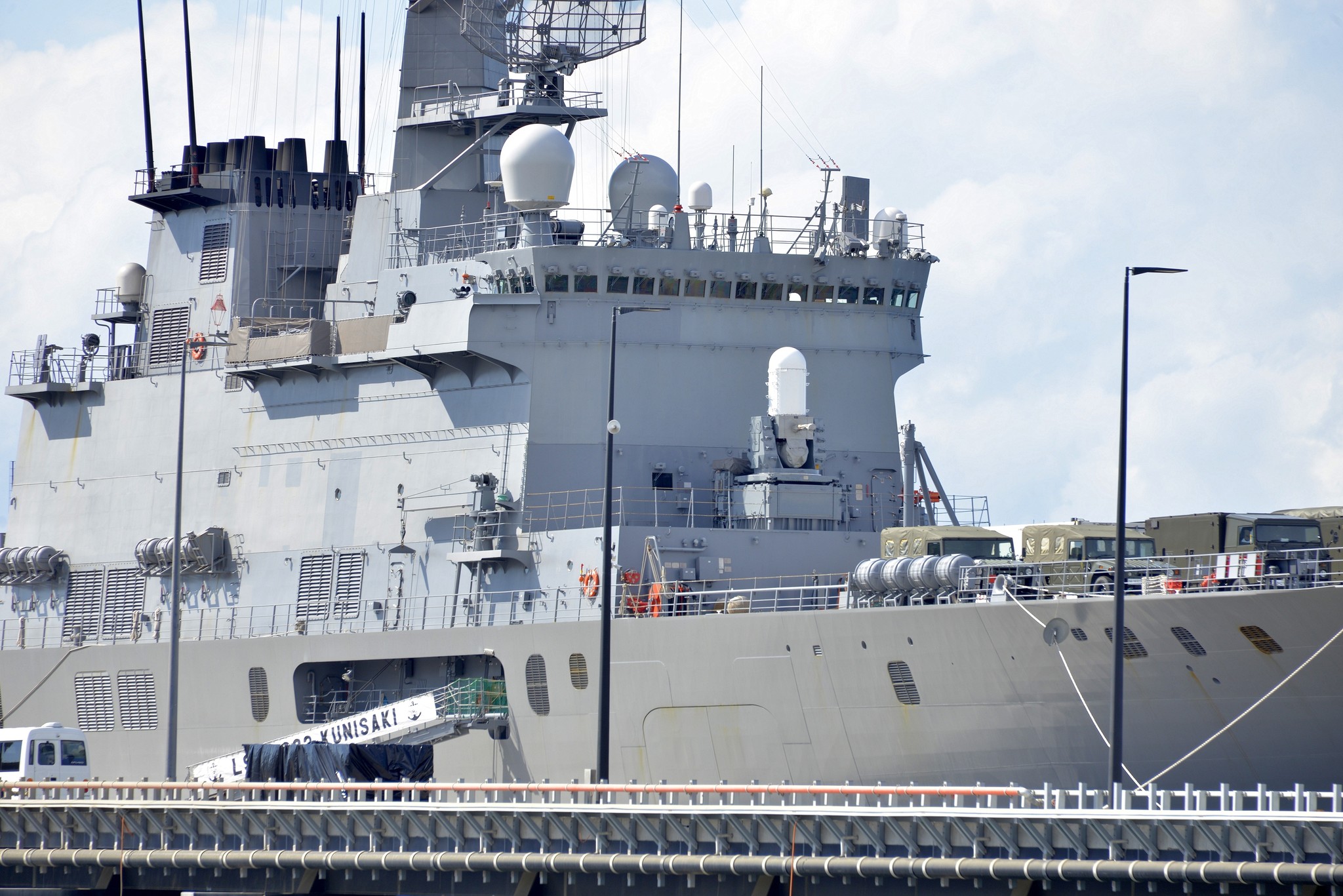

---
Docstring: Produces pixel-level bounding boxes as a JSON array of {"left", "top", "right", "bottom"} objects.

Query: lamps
[
  {"left": 607, "top": 236, "right": 618, "bottom": 247},
  {"left": 342, "top": 669, "right": 354, "bottom": 681},
  {"left": 908, "top": 248, "right": 940, "bottom": 263},
  {"left": 620, "top": 237, "right": 631, "bottom": 247}
]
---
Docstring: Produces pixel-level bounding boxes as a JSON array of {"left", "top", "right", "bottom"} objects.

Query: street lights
[
  {"left": 595, "top": 306, "right": 673, "bottom": 786},
  {"left": 1108, "top": 265, "right": 1192, "bottom": 807},
  {"left": 165, "top": 340, "right": 238, "bottom": 781}
]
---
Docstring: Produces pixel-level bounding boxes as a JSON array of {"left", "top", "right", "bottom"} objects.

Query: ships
[{"left": 0, "top": 0, "right": 1343, "bottom": 791}]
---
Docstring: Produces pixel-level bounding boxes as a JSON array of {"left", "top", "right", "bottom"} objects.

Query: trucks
[
  {"left": 1022, "top": 524, "right": 1182, "bottom": 598},
  {"left": 880, "top": 526, "right": 1040, "bottom": 594},
  {"left": 1143, "top": 512, "right": 1332, "bottom": 586}
]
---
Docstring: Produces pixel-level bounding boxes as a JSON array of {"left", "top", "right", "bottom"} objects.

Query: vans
[{"left": 0, "top": 721, "right": 94, "bottom": 804}]
